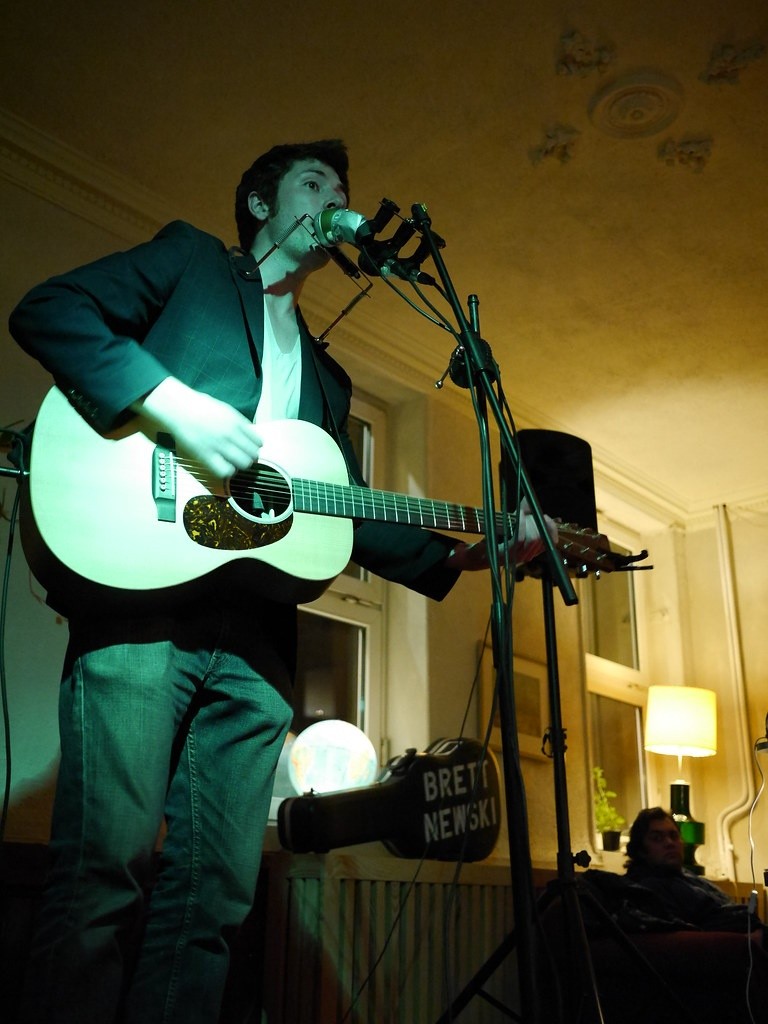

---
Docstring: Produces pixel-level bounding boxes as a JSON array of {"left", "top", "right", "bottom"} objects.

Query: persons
[
  {"left": 7, "top": 138, "right": 563, "bottom": 1024},
  {"left": 594, "top": 806, "right": 768, "bottom": 953}
]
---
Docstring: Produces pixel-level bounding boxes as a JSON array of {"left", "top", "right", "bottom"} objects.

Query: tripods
[{"left": 438, "top": 583, "right": 694, "bottom": 1024}]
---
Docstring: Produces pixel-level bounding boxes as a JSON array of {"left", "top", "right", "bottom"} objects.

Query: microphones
[
  {"left": 451, "top": 338, "right": 500, "bottom": 389},
  {"left": 358, "top": 252, "right": 436, "bottom": 287},
  {"left": 315, "top": 209, "right": 374, "bottom": 251}
]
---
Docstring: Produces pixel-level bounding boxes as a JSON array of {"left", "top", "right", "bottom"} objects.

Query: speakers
[{"left": 499, "top": 429, "right": 599, "bottom": 583}]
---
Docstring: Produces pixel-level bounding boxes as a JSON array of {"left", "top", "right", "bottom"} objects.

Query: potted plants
[{"left": 593, "top": 766, "right": 626, "bottom": 852}]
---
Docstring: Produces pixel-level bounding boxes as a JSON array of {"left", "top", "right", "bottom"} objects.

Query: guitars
[{"left": 18, "top": 379, "right": 633, "bottom": 616}]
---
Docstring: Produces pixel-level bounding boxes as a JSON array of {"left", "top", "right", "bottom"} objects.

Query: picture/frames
[{"left": 475, "top": 638, "right": 552, "bottom": 765}]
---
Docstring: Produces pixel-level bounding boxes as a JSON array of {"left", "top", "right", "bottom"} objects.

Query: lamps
[{"left": 643, "top": 684, "right": 718, "bottom": 876}]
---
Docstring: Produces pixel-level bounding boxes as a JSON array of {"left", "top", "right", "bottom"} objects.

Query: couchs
[{"left": 532, "top": 900, "right": 768, "bottom": 1024}]
